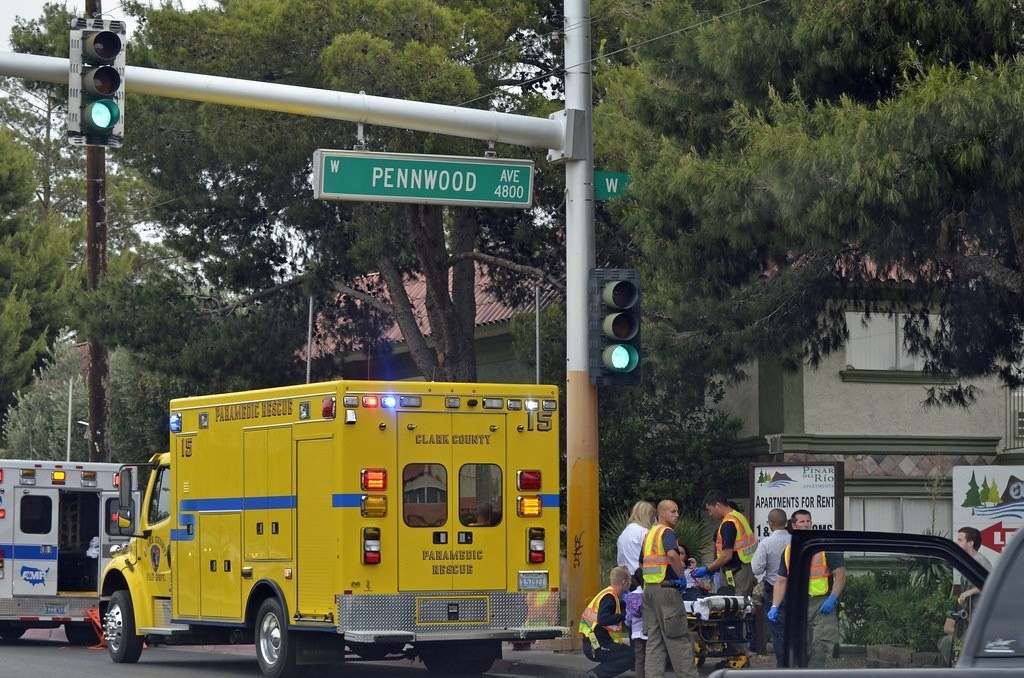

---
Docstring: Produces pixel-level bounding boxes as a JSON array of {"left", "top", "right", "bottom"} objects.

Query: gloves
[
  {"left": 767, "top": 607, "right": 779, "bottom": 622},
  {"left": 691, "top": 567, "right": 708, "bottom": 578},
  {"left": 670, "top": 576, "right": 687, "bottom": 594},
  {"left": 819, "top": 594, "right": 837, "bottom": 614}
]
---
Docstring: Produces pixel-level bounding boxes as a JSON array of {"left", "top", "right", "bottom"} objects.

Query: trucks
[{"left": 0, "top": 459, "right": 141, "bottom": 647}]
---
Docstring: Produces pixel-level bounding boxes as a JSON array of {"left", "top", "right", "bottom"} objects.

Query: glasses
[{"left": 766, "top": 520, "right": 774, "bottom": 523}]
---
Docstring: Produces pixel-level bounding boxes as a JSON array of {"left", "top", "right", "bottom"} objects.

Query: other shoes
[
  {"left": 586, "top": 669, "right": 599, "bottom": 678},
  {"left": 716, "top": 657, "right": 750, "bottom": 669}
]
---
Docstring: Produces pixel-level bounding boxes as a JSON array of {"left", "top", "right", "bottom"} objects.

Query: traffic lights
[
  {"left": 590, "top": 269, "right": 642, "bottom": 387},
  {"left": 67, "top": 17, "right": 125, "bottom": 148}
]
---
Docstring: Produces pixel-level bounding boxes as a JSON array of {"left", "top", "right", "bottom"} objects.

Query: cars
[{"left": 782, "top": 509, "right": 1024, "bottom": 677}]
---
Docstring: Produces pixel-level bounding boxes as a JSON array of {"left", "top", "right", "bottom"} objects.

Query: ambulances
[{"left": 99, "top": 381, "right": 569, "bottom": 677}]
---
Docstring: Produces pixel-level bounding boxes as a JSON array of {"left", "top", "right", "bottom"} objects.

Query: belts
[{"left": 661, "top": 584, "right": 678, "bottom": 589}]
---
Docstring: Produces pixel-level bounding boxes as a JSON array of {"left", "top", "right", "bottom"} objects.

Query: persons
[
  {"left": 579, "top": 500, "right": 731, "bottom": 678},
  {"left": 579, "top": 565, "right": 634, "bottom": 678},
  {"left": 751, "top": 509, "right": 792, "bottom": 667},
  {"left": 768, "top": 510, "right": 845, "bottom": 670},
  {"left": 639, "top": 500, "right": 699, "bottom": 677},
  {"left": 957, "top": 527, "right": 992, "bottom": 604},
  {"left": 692, "top": 491, "right": 757, "bottom": 667}
]
